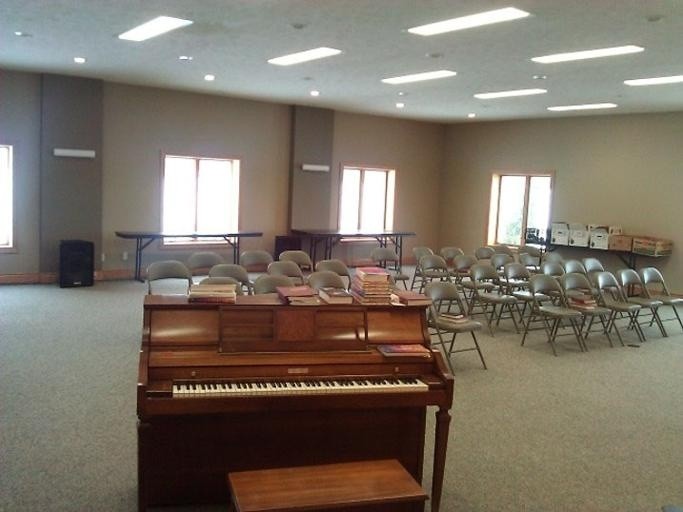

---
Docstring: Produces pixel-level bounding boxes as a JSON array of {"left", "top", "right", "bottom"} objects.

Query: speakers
[
  {"left": 274, "top": 236, "right": 299, "bottom": 260},
  {"left": 60, "top": 240, "right": 94, "bottom": 286}
]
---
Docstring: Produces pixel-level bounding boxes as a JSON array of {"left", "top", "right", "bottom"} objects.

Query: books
[
  {"left": 570, "top": 295, "right": 598, "bottom": 310},
  {"left": 395, "top": 290, "right": 433, "bottom": 306},
  {"left": 187, "top": 297, "right": 236, "bottom": 304},
  {"left": 317, "top": 286, "right": 353, "bottom": 305},
  {"left": 376, "top": 343, "right": 431, "bottom": 358},
  {"left": 348, "top": 264, "right": 393, "bottom": 307},
  {"left": 278, "top": 293, "right": 319, "bottom": 306},
  {"left": 438, "top": 316, "right": 468, "bottom": 325},
  {"left": 189, "top": 283, "right": 237, "bottom": 292},
  {"left": 188, "top": 291, "right": 237, "bottom": 298},
  {"left": 276, "top": 284, "right": 317, "bottom": 300},
  {"left": 441, "top": 311, "right": 463, "bottom": 319}
]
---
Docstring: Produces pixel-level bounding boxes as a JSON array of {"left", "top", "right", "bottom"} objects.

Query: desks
[
  {"left": 115, "top": 231, "right": 263, "bottom": 281},
  {"left": 291, "top": 228, "right": 417, "bottom": 274}
]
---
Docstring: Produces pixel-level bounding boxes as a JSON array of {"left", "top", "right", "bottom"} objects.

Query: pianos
[{"left": 137, "top": 294, "right": 453, "bottom": 512}]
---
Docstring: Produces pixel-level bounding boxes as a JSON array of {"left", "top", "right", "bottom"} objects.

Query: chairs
[
  {"left": 370, "top": 247, "right": 409, "bottom": 290},
  {"left": 424, "top": 281, "right": 489, "bottom": 374},
  {"left": 411, "top": 244, "right": 682, "bottom": 357},
  {"left": 147, "top": 242, "right": 351, "bottom": 296}
]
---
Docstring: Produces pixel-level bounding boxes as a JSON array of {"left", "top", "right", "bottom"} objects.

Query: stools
[{"left": 228, "top": 457, "right": 429, "bottom": 511}]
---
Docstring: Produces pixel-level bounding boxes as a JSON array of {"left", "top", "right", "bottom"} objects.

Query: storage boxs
[{"left": 549, "top": 221, "right": 673, "bottom": 257}]
